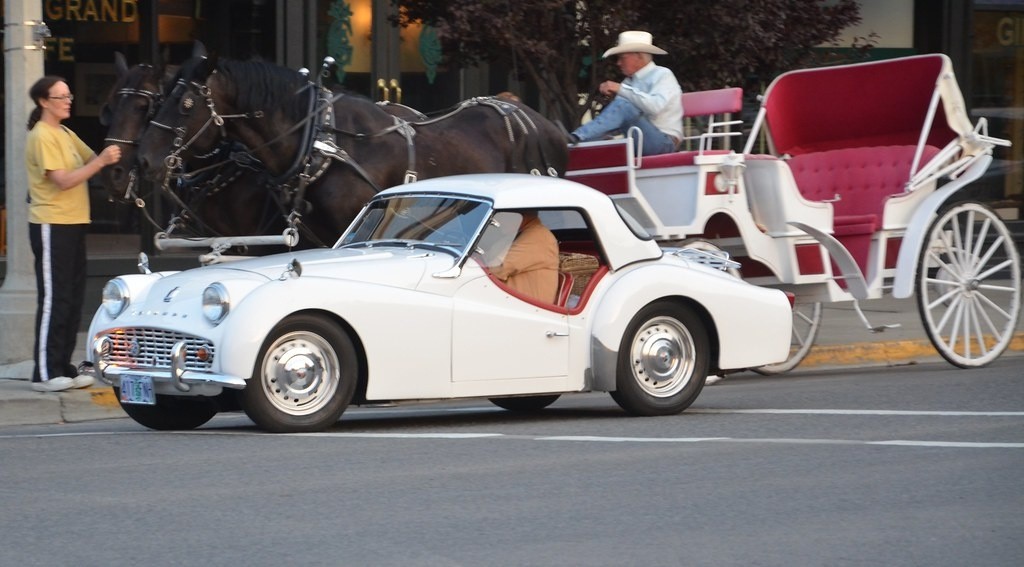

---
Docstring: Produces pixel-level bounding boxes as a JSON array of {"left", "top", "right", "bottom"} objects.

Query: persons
[
  {"left": 485, "top": 208, "right": 560, "bottom": 306},
  {"left": 24, "top": 76, "right": 121, "bottom": 392},
  {"left": 553, "top": 31, "right": 684, "bottom": 154}
]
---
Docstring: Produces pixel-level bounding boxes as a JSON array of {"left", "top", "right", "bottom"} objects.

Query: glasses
[{"left": 46, "top": 94, "right": 73, "bottom": 102}]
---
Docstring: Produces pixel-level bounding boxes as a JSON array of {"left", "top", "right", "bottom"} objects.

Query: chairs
[{"left": 553, "top": 270, "right": 575, "bottom": 309}]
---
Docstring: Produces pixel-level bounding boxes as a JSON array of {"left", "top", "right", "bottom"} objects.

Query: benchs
[
  {"left": 740, "top": 152, "right": 844, "bottom": 267},
  {"left": 634, "top": 88, "right": 744, "bottom": 218},
  {"left": 787, "top": 144, "right": 943, "bottom": 279}
]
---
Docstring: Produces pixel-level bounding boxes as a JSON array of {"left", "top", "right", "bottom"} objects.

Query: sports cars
[{"left": 88, "top": 174, "right": 795, "bottom": 444}]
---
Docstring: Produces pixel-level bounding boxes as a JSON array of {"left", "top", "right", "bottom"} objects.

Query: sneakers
[
  {"left": 32, "top": 376, "right": 73, "bottom": 391},
  {"left": 71, "top": 375, "right": 94, "bottom": 389}
]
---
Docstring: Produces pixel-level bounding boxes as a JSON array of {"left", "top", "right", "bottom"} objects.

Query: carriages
[{"left": 95, "top": 41, "right": 1024, "bottom": 375}]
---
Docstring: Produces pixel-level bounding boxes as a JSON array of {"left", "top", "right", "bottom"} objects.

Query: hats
[{"left": 602, "top": 30, "right": 668, "bottom": 58}]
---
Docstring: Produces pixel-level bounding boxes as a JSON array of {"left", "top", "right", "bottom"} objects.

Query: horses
[{"left": 103, "top": 35, "right": 570, "bottom": 254}]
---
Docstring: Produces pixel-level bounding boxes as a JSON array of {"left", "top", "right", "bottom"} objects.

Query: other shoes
[{"left": 552, "top": 119, "right": 577, "bottom": 144}]
